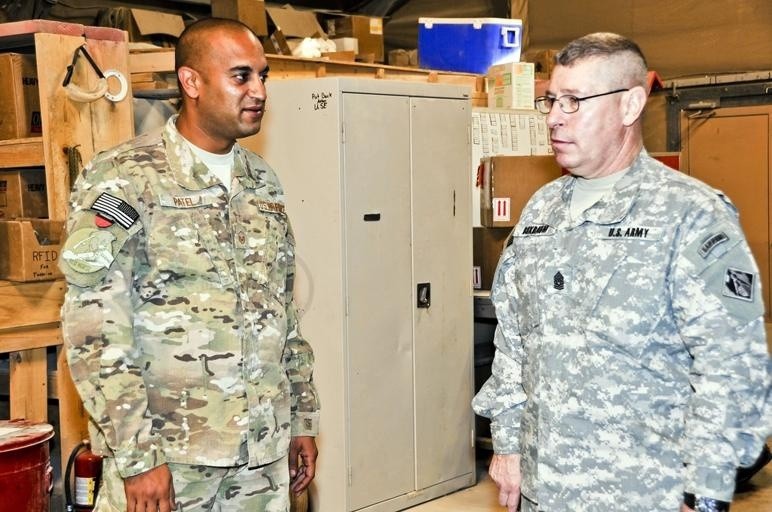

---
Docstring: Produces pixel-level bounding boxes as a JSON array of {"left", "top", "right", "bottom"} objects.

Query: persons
[
  {"left": 59, "top": 16, "right": 320, "bottom": 511},
  {"left": 471, "top": 30, "right": 772, "bottom": 510}
]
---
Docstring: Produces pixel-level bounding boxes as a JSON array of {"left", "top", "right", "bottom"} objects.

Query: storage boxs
[
  {"left": 476, "top": 228, "right": 515, "bottom": 288},
  {"left": 95, "top": 7, "right": 185, "bottom": 42},
  {"left": 1, "top": 170, "right": 47, "bottom": 218},
  {"left": 0, "top": 52, "right": 42, "bottom": 139},
  {"left": 479, "top": 156, "right": 563, "bottom": 228},
  {"left": 2, "top": 221, "right": 69, "bottom": 282},
  {"left": 417, "top": 18, "right": 523, "bottom": 75}
]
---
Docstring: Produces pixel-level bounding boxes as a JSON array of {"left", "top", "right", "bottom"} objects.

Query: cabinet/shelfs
[
  {"left": 256, "top": 80, "right": 477, "bottom": 512},
  {"left": 677, "top": 107, "right": 771, "bottom": 322},
  {"left": 0, "top": 19, "right": 135, "bottom": 490}
]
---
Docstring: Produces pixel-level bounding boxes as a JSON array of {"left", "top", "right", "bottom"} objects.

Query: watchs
[{"left": 683, "top": 492, "right": 730, "bottom": 512}]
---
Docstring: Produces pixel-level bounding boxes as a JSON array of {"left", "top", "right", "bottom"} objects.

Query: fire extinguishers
[{"left": 63, "top": 437, "right": 102, "bottom": 511}]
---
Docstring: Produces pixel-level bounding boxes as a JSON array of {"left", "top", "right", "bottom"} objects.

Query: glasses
[{"left": 535, "top": 86, "right": 627, "bottom": 114}]
